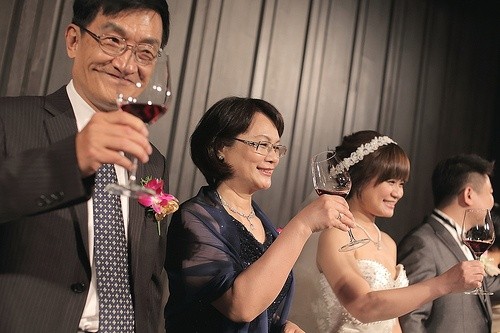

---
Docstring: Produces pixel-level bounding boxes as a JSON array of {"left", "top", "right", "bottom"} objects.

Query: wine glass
[
  {"left": 312, "top": 151, "right": 371, "bottom": 252},
  {"left": 107, "top": 45, "right": 172, "bottom": 199},
  {"left": 461, "top": 210, "right": 496, "bottom": 295}
]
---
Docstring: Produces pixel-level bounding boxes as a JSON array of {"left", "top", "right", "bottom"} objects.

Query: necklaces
[
  {"left": 354, "top": 222, "right": 383, "bottom": 250},
  {"left": 218, "top": 194, "right": 256, "bottom": 231}
]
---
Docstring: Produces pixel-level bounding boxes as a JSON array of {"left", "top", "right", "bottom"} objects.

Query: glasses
[
  {"left": 83, "top": 27, "right": 163, "bottom": 67},
  {"left": 234, "top": 137, "right": 289, "bottom": 159}
]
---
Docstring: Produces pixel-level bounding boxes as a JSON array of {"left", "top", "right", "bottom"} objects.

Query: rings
[{"left": 337, "top": 212, "right": 343, "bottom": 219}]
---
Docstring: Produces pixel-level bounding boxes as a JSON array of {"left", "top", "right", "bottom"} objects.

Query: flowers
[
  {"left": 140, "top": 174, "right": 179, "bottom": 236},
  {"left": 275, "top": 223, "right": 284, "bottom": 233}
]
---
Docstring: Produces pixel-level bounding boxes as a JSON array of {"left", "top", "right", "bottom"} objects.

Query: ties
[{"left": 93, "top": 162, "right": 136, "bottom": 333}]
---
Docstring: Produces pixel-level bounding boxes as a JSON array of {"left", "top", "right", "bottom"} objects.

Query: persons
[
  {"left": 0, "top": 0, "right": 170, "bottom": 333},
  {"left": 396, "top": 154, "right": 500, "bottom": 333},
  {"left": 315, "top": 130, "right": 484, "bottom": 333},
  {"left": 165, "top": 97, "right": 356, "bottom": 333}
]
